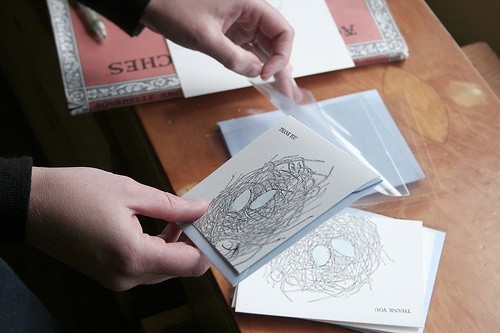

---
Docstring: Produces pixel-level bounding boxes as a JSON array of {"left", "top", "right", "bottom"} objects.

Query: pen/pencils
[{"left": 72, "top": 0, "right": 109, "bottom": 41}]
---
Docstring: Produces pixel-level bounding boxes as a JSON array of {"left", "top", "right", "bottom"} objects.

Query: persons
[{"left": 0, "top": 0, "right": 304, "bottom": 332}]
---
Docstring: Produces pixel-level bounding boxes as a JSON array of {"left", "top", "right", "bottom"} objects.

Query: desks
[{"left": 99, "top": 0, "right": 499, "bottom": 333}]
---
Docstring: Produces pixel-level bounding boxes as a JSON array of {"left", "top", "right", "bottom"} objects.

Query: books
[{"left": 45, "top": 0, "right": 412, "bottom": 117}]
